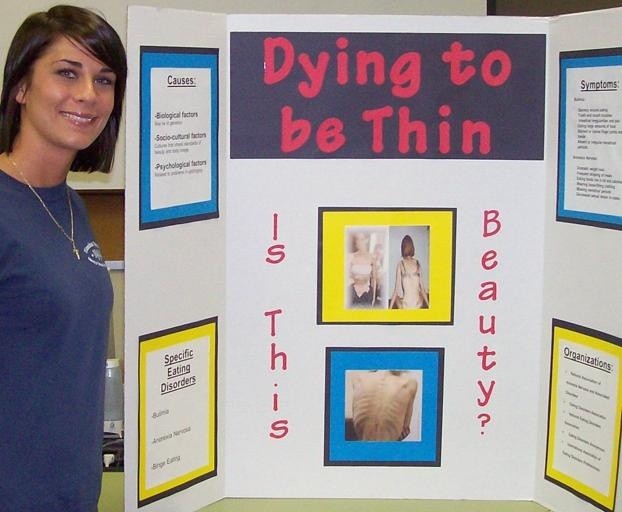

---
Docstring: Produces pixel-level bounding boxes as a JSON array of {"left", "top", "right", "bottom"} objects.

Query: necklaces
[{"left": 10, "top": 155, "right": 82, "bottom": 260}]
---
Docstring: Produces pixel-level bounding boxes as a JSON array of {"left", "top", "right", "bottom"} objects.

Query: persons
[
  {"left": 1, "top": 4, "right": 128, "bottom": 511},
  {"left": 346, "top": 230, "right": 387, "bottom": 310},
  {"left": 389, "top": 235, "right": 429, "bottom": 310},
  {"left": 350, "top": 368, "right": 418, "bottom": 442}
]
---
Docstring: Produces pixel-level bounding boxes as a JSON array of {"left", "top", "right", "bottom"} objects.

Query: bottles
[{"left": 101, "top": 358, "right": 122, "bottom": 438}]
